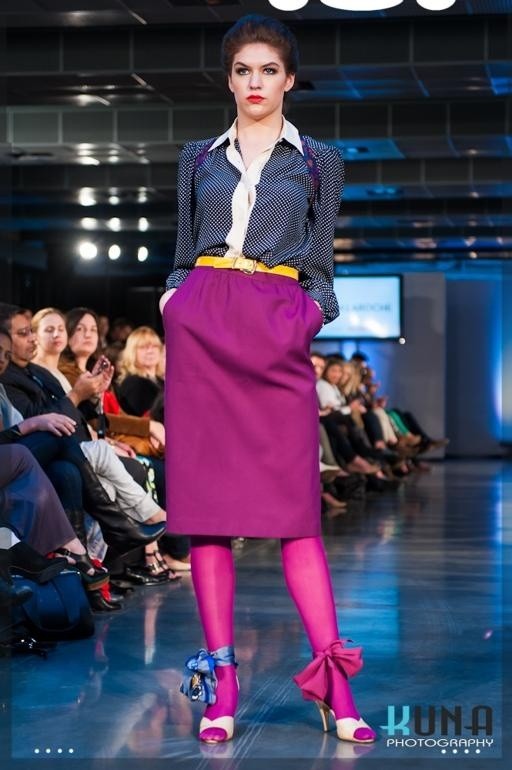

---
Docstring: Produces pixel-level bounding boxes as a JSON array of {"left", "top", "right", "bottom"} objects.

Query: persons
[
  {"left": 158, "top": 15, "right": 377, "bottom": 745},
  {"left": 2, "top": 307, "right": 452, "bottom": 659}
]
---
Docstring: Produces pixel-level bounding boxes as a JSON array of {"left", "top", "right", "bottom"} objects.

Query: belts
[{"left": 195, "top": 254, "right": 300, "bottom": 280}]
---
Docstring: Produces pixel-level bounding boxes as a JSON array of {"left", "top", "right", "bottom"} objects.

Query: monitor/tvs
[{"left": 313, "top": 273, "right": 406, "bottom": 343}]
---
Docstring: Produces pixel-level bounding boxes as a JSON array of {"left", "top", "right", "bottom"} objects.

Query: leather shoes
[{"left": 0, "top": 510, "right": 197, "bottom": 659}]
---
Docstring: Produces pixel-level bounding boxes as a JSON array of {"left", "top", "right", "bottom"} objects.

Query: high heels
[
  {"left": 196, "top": 671, "right": 242, "bottom": 746},
  {"left": 320, "top": 433, "right": 451, "bottom": 511},
  {"left": 310, "top": 688, "right": 377, "bottom": 745}
]
